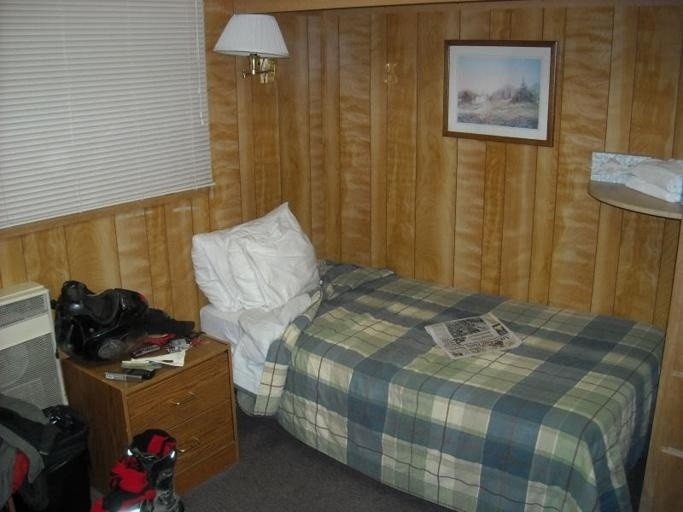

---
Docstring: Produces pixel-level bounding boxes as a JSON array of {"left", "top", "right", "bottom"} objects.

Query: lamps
[{"left": 212, "top": 14, "right": 289, "bottom": 84}]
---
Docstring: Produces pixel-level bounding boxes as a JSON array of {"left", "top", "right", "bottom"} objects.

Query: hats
[{"left": 145, "top": 308, "right": 194, "bottom": 334}]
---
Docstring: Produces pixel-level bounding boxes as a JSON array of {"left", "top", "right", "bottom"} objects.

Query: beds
[{"left": 199, "top": 257, "right": 665, "bottom": 512}]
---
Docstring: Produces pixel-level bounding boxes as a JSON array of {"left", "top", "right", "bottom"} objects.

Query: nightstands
[{"left": 63, "top": 346, "right": 240, "bottom": 499}]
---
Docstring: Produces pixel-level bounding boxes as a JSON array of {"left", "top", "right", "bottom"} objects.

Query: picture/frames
[{"left": 443, "top": 38, "right": 559, "bottom": 147}]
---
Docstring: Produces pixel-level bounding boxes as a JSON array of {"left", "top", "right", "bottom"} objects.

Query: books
[{"left": 104, "top": 360, "right": 162, "bottom": 382}]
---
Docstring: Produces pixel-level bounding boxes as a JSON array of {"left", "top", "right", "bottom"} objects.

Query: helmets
[{"left": 52, "top": 281, "right": 146, "bottom": 361}]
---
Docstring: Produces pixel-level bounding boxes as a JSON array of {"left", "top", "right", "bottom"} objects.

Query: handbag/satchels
[{"left": 12, "top": 404, "right": 90, "bottom": 512}]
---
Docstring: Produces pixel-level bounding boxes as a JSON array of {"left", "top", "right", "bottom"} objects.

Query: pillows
[{"left": 192, "top": 202, "right": 322, "bottom": 312}]
